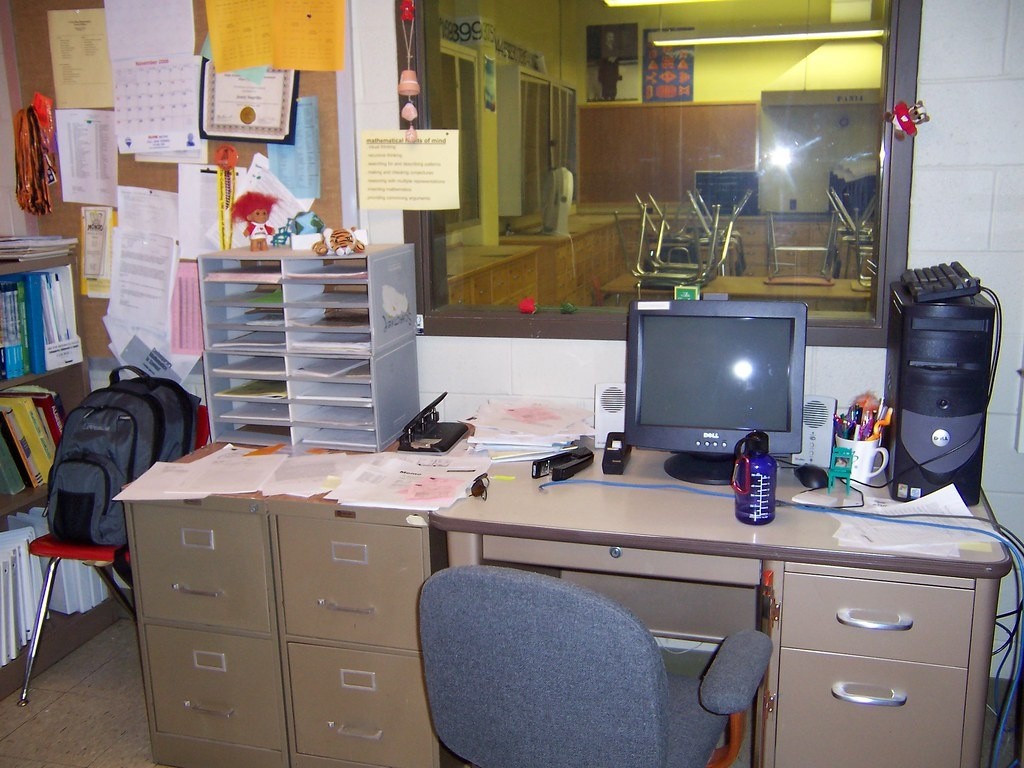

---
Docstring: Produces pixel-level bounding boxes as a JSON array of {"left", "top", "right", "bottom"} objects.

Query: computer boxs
[{"left": 882, "top": 279, "right": 996, "bottom": 506}]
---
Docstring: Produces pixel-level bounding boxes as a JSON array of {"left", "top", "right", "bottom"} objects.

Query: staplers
[{"left": 532, "top": 446, "right": 595, "bottom": 481}]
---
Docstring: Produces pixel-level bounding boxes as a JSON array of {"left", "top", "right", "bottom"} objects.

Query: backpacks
[{"left": 42, "top": 366, "right": 201, "bottom": 546}]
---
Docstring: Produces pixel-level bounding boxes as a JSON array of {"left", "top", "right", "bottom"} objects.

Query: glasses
[{"left": 468, "top": 474, "right": 490, "bottom": 501}]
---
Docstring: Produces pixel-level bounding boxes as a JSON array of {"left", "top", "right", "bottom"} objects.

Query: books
[
  {"left": 164, "top": 454, "right": 288, "bottom": 494},
  {"left": 0, "top": 507, "right": 109, "bottom": 670},
  {"left": 0, "top": 234, "right": 78, "bottom": 261},
  {"left": 214, "top": 379, "right": 288, "bottom": 400},
  {"left": 0, "top": 264, "right": 77, "bottom": 380},
  {"left": 0, "top": 386, "right": 66, "bottom": 495},
  {"left": 294, "top": 358, "right": 369, "bottom": 377}
]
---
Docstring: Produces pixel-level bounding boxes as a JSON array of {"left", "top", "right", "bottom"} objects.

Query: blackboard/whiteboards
[
  {"left": 756, "top": 104, "right": 882, "bottom": 214},
  {"left": 694, "top": 170, "right": 758, "bottom": 216}
]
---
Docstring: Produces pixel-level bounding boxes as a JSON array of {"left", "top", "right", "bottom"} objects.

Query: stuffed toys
[{"left": 313, "top": 226, "right": 365, "bottom": 255}]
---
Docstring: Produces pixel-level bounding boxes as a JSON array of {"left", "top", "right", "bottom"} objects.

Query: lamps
[{"left": 648, "top": 0, "right": 887, "bottom": 47}]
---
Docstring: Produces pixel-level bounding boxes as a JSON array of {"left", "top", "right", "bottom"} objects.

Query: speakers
[
  {"left": 594, "top": 384, "right": 626, "bottom": 448},
  {"left": 791, "top": 396, "right": 838, "bottom": 469}
]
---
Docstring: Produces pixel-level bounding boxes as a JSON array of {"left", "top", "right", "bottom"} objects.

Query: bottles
[{"left": 732, "top": 430, "right": 776, "bottom": 524}]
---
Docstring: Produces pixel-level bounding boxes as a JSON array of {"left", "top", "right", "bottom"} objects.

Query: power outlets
[{"left": 789, "top": 199, "right": 797, "bottom": 210}]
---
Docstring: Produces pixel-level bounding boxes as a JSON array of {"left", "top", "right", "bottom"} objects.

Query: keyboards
[{"left": 901, "top": 261, "right": 981, "bottom": 303}]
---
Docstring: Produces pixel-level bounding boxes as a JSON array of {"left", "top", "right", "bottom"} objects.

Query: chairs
[
  {"left": 18, "top": 405, "right": 210, "bottom": 706},
  {"left": 766, "top": 186, "right": 878, "bottom": 280},
  {"left": 417, "top": 565, "right": 774, "bottom": 768},
  {"left": 612, "top": 189, "right": 754, "bottom": 292}
]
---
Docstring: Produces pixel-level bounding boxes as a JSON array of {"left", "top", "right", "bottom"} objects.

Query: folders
[
  {"left": 0, "top": 506, "right": 109, "bottom": 666},
  {"left": 212, "top": 380, "right": 287, "bottom": 400}
]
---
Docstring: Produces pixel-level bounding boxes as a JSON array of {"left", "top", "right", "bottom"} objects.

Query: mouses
[{"left": 794, "top": 462, "right": 829, "bottom": 488}]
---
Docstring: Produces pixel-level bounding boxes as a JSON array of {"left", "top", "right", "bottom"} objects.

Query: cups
[{"left": 835, "top": 435, "right": 889, "bottom": 487}]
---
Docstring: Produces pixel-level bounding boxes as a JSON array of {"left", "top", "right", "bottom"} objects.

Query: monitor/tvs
[
  {"left": 695, "top": 171, "right": 759, "bottom": 217},
  {"left": 624, "top": 301, "right": 807, "bottom": 486}
]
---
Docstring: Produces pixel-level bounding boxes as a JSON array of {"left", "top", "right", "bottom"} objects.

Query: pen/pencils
[{"left": 833, "top": 397, "right": 888, "bottom": 446}]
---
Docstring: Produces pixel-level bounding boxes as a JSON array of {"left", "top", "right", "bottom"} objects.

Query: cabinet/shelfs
[
  {"left": 0, "top": 256, "right": 118, "bottom": 703},
  {"left": 618, "top": 214, "right": 875, "bottom": 279},
  {"left": 446, "top": 243, "right": 542, "bottom": 308},
  {"left": 119, "top": 423, "right": 1012, "bottom": 768},
  {"left": 498, "top": 213, "right": 624, "bottom": 307},
  {"left": 196, "top": 241, "right": 420, "bottom": 453}
]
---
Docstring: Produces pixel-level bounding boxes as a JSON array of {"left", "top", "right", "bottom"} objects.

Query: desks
[{"left": 601, "top": 271, "right": 872, "bottom": 312}]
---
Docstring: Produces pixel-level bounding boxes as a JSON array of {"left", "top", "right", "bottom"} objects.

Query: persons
[
  {"left": 597, "top": 31, "right": 620, "bottom": 100},
  {"left": 230, "top": 192, "right": 275, "bottom": 251}
]
